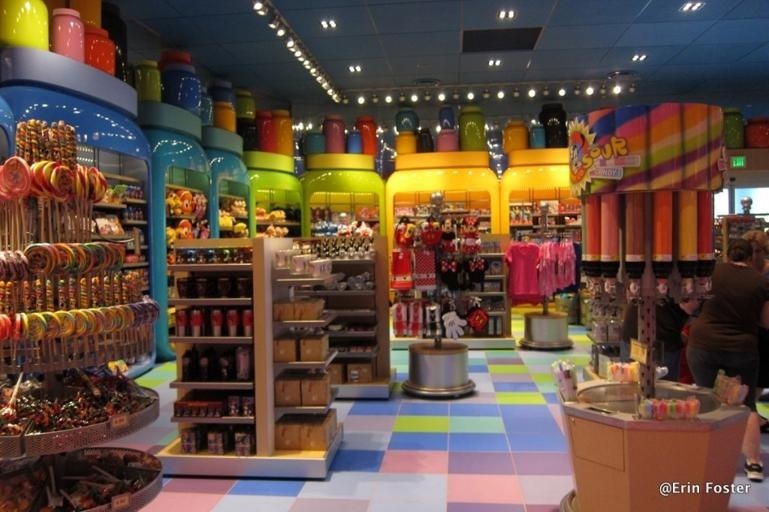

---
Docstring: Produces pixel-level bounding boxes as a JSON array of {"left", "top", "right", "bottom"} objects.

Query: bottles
[
  {"left": 723, "top": 106, "right": 769, "bottom": 148},
  {"left": 300, "top": 101, "right": 570, "bottom": 157},
  {"left": 0, "top": 0, "right": 295, "bottom": 157},
  {"left": 173, "top": 307, "right": 252, "bottom": 382}
]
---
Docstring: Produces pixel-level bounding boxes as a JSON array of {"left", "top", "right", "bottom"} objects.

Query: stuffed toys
[
  {"left": 312, "top": 188, "right": 506, "bottom": 339},
  {"left": 162, "top": 186, "right": 303, "bottom": 254}
]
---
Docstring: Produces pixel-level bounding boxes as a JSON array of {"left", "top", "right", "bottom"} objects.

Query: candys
[
  {"left": 638, "top": 395, "right": 700, "bottom": 419},
  {"left": 606, "top": 363, "right": 640, "bottom": 382},
  {"left": 0, "top": 118, "right": 161, "bottom": 510}
]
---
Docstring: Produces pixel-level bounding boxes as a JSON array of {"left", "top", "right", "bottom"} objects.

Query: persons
[{"left": 618, "top": 219, "right": 769, "bottom": 483}]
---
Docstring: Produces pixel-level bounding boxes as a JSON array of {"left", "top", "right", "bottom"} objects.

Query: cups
[{"left": 174, "top": 276, "right": 247, "bottom": 299}]
